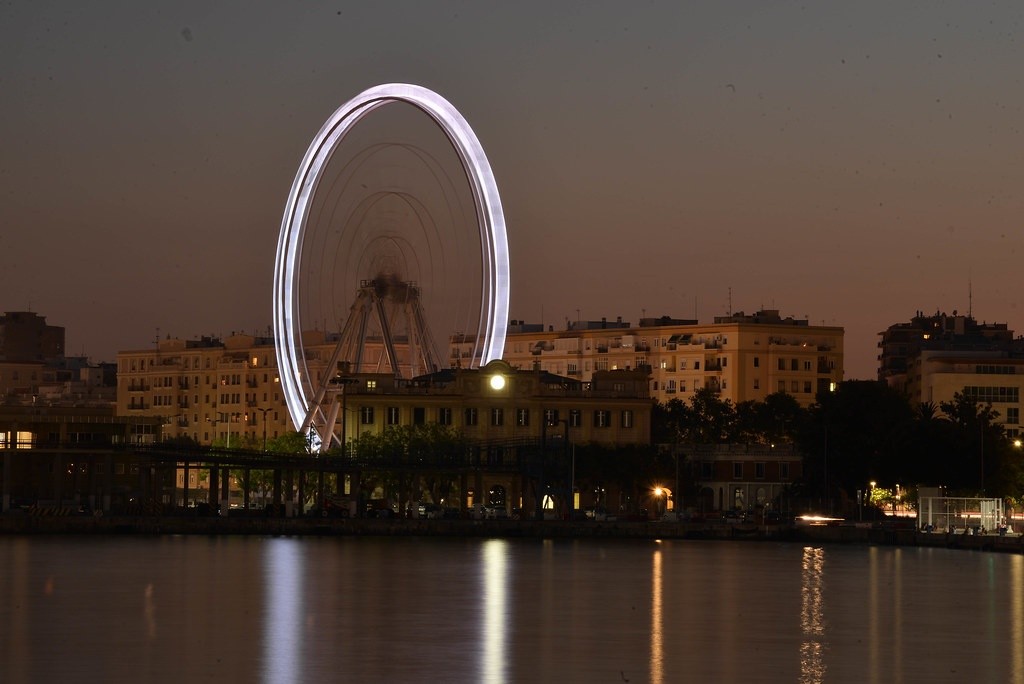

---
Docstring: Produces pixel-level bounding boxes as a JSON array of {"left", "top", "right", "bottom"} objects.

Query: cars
[
  {"left": 392, "top": 498, "right": 444, "bottom": 519},
  {"left": 466, "top": 503, "right": 507, "bottom": 520},
  {"left": 662, "top": 504, "right": 705, "bottom": 522}
]
